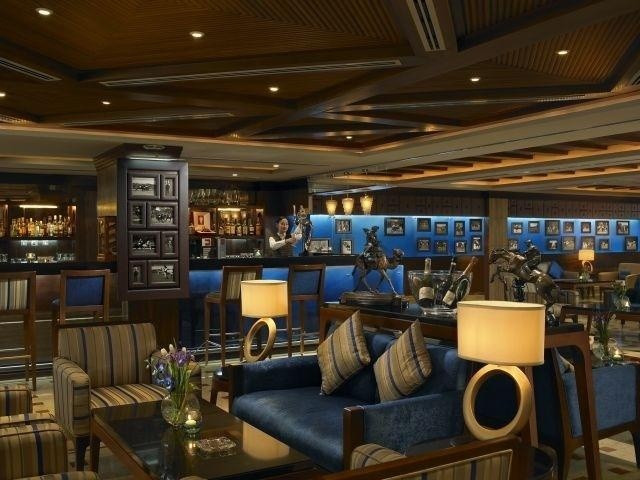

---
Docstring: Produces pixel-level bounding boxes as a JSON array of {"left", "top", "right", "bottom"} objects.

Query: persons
[{"left": 269, "top": 217, "right": 299, "bottom": 262}]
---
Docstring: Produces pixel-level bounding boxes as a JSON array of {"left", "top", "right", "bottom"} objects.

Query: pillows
[
  {"left": 373, "top": 318, "right": 433, "bottom": 402},
  {"left": 618, "top": 269, "right": 631, "bottom": 280},
  {"left": 350, "top": 443, "right": 408, "bottom": 471},
  {"left": 315, "top": 309, "right": 371, "bottom": 396},
  {"left": 548, "top": 261, "right": 565, "bottom": 279}
]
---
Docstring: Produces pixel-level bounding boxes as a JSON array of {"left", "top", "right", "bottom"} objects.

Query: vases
[{"left": 161, "top": 393, "right": 201, "bottom": 427}]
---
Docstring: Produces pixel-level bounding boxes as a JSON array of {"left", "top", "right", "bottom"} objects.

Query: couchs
[
  {"left": 608, "top": 275, "right": 640, "bottom": 311},
  {"left": 531, "top": 322, "right": 640, "bottom": 470},
  {"left": 52, "top": 322, "right": 171, "bottom": 472},
  {"left": 227, "top": 309, "right": 539, "bottom": 470},
  {"left": 0, "top": 383, "right": 100, "bottom": 480},
  {"left": 536, "top": 261, "right": 580, "bottom": 301},
  {"left": 598, "top": 262, "right": 640, "bottom": 289}
]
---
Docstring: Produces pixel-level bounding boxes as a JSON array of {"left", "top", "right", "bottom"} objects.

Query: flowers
[{"left": 144, "top": 339, "right": 222, "bottom": 396}]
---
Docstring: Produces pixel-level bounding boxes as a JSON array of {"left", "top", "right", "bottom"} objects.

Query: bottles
[
  {"left": 436, "top": 258, "right": 457, "bottom": 306},
  {"left": 236, "top": 217, "right": 242, "bottom": 236},
  {"left": 220, "top": 218, "right": 222, "bottom": 226},
  {"left": 219, "top": 226, "right": 225, "bottom": 235},
  {"left": 226, "top": 217, "right": 230, "bottom": 235},
  {"left": 442, "top": 255, "right": 479, "bottom": 308},
  {"left": 10, "top": 215, "right": 72, "bottom": 237},
  {"left": 419, "top": 257, "right": 436, "bottom": 307},
  {"left": 222, "top": 220, "right": 225, "bottom": 229},
  {"left": 255, "top": 216, "right": 262, "bottom": 235},
  {"left": 242, "top": 218, "right": 248, "bottom": 235},
  {"left": 230, "top": 217, "right": 235, "bottom": 236},
  {"left": 249, "top": 218, "right": 255, "bottom": 235}
]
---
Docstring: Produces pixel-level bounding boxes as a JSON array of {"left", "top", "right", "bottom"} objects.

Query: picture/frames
[
  {"left": 625, "top": 236, "right": 638, "bottom": 251},
  {"left": 129, "top": 261, "right": 147, "bottom": 289},
  {"left": 384, "top": 217, "right": 405, "bottom": 236},
  {"left": 161, "top": 231, "right": 178, "bottom": 258},
  {"left": 340, "top": 239, "right": 354, "bottom": 255},
  {"left": 434, "top": 222, "right": 448, "bottom": 235},
  {"left": 416, "top": 237, "right": 431, "bottom": 252},
  {"left": 127, "top": 200, "right": 146, "bottom": 229},
  {"left": 188, "top": 208, "right": 218, "bottom": 235},
  {"left": 308, "top": 238, "right": 332, "bottom": 254},
  {"left": 509, "top": 240, "right": 519, "bottom": 251},
  {"left": 471, "top": 235, "right": 482, "bottom": 252},
  {"left": 455, "top": 240, "right": 467, "bottom": 254},
  {"left": 616, "top": 221, "right": 630, "bottom": 235},
  {"left": 434, "top": 240, "right": 448, "bottom": 254},
  {"left": 128, "top": 231, "right": 160, "bottom": 260},
  {"left": 161, "top": 174, "right": 178, "bottom": 200},
  {"left": 595, "top": 221, "right": 609, "bottom": 236},
  {"left": 116, "top": 157, "right": 191, "bottom": 302},
  {"left": 545, "top": 220, "right": 561, "bottom": 236},
  {"left": 454, "top": 221, "right": 465, "bottom": 237},
  {"left": 417, "top": 218, "right": 431, "bottom": 232},
  {"left": 581, "top": 222, "right": 591, "bottom": 233},
  {"left": 147, "top": 201, "right": 178, "bottom": 229},
  {"left": 469, "top": 219, "right": 482, "bottom": 232},
  {"left": 561, "top": 236, "right": 576, "bottom": 251},
  {"left": 528, "top": 221, "right": 540, "bottom": 233},
  {"left": 127, "top": 172, "right": 161, "bottom": 200},
  {"left": 147, "top": 260, "right": 179, "bottom": 288},
  {"left": 547, "top": 239, "right": 558, "bottom": 250},
  {"left": 599, "top": 239, "right": 609, "bottom": 250},
  {"left": 582, "top": 236, "right": 595, "bottom": 250},
  {"left": 335, "top": 218, "right": 352, "bottom": 234},
  {"left": 563, "top": 222, "right": 574, "bottom": 233},
  {"left": 511, "top": 223, "right": 522, "bottom": 235}
]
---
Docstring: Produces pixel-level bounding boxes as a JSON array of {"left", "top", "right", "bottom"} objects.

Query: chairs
[
  {"left": 51, "top": 267, "right": 111, "bottom": 358},
  {"left": 203, "top": 264, "right": 264, "bottom": 367},
  {"left": 0, "top": 270, "right": 36, "bottom": 391},
  {"left": 269, "top": 264, "right": 327, "bottom": 358}
]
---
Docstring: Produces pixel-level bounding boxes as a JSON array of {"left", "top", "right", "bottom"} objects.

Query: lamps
[
  {"left": 325, "top": 199, "right": 337, "bottom": 216},
  {"left": 578, "top": 249, "right": 594, "bottom": 261},
  {"left": 360, "top": 196, "right": 374, "bottom": 216},
  {"left": 240, "top": 279, "right": 289, "bottom": 318},
  {"left": 456, "top": 299, "right": 546, "bottom": 367},
  {"left": 340, "top": 198, "right": 354, "bottom": 215},
  {"left": 17, "top": 204, "right": 61, "bottom": 209}
]
